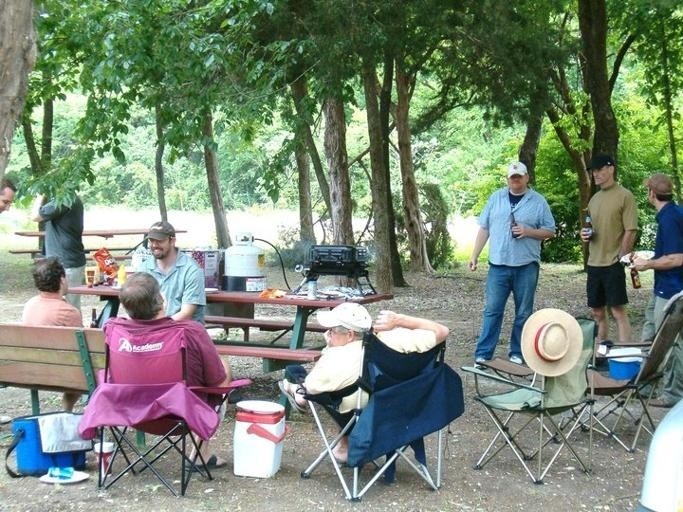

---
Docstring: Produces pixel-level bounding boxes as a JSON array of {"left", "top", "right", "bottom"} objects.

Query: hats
[
  {"left": 586, "top": 155, "right": 615, "bottom": 171},
  {"left": 643, "top": 175, "right": 673, "bottom": 196},
  {"left": 317, "top": 302, "right": 372, "bottom": 333},
  {"left": 506, "top": 162, "right": 527, "bottom": 179},
  {"left": 520, "top": 307, "right": 584, "bottom": 378},
  {"left": 144, "top": 221, "right": 175, "bottom": 242}
]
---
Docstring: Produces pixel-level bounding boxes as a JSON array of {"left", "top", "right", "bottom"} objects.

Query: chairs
[
  {"left": 287, "top": 328, "right": 465, "bottom": 502},
  {"left": 572, "top": 290, "right": 683, "bottom": 453},
  {"left": 78, "top": 324, "right": 254, "bottom": 499},
  {"left": 459, "top": 318, "right": 598, "bottom": 484}
]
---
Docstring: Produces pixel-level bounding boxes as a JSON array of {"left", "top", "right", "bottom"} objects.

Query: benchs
[
  {"left": 34, "top": 255, "right": 132, "bottom": 264},
  {"left": 202, "top": 313, "right": 331, "bottom": 344},
  {"left": 204, "top": 315, "right": 343, "bottom": 332},
  {"left": 0, "top": 323, "right": 148, "bottom": 449},
  {"left": 9, "top": 245, "right": 137, "bottom": 256}
]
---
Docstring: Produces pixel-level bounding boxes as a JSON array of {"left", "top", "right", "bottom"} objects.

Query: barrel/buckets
[{"left": 233, "top": 401, "right": 288, "bottom": 478}]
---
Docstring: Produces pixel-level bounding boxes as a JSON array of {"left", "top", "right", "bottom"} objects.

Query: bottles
[
  {"left": 510, "top": 213, "right": 519, "bottom": 238},
  {"left": 582, "top": 207, "right": 595, "bottom": 240},
  {"left": 118, "top": 262, "right": 128, "bottom": 285},
  {"left": 307, "top": 278, "right": 318, "bottom": 300},
  {"left": 628, "top": 255, "right": 642, "bottom": 289},
  {"left": 84, "top": 259, "right": 100, "bottom": 286}
]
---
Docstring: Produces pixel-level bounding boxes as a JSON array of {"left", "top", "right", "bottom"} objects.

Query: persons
[
  {"left": 628, "top": 174, "right": 683, "bottom": 408},
  {"left": 21, "top": 256, "right": 86, "bottom": 412},
  {"left": 278, "top": 303, "right": 451, "bottom": 464},
  {"left": 0, "top": 177, "right": 16, "bottom": 423},
  {"left": 30, "top": 178, "right": 84, "bottom": 312},
  {"left": 126, "top": 222, "right": 207, "bottom": 328},
  {"left": 469, "top": 161, "right": 556, "bottom": 370},
  {"left": 99, "top": 273, "right": 232, "bottom": 472},
  {"left": 636, "top": 399, "right": 682, "bottom": 512},
  {"left": 580, "top": 153, "right": 638, "bottom": 355}
]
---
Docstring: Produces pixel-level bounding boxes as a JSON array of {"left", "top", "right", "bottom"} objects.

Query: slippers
[
  {"left": 185, "top": 455, "right": 226, "bottom": 472},
  {"left": 278, "top": 378, "right": 310, "bottom": 415}
]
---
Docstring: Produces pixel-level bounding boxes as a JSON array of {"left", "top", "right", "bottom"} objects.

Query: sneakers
[
  {"left": 648, "top": 396, "right": 674, "bottom": 407},
  {"left": 474, "top": 357, "right": 486, "bottom": 369},
  {"left": 510, "top": 354, "right": 523, "bottom": 366}
]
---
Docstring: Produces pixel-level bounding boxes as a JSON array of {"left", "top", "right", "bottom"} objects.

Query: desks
[
  {"left": 14, "top": 229, "right": 187, "bottom": 256},
  {"left": 68, "top": 284, "right": 393, "bottom": 420}
]
